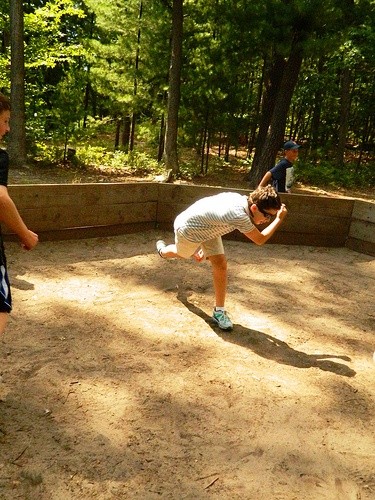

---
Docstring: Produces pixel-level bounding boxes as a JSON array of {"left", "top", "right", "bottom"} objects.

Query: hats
[{"left": 283, "top": 141, "right": 300, "bottom": 150}]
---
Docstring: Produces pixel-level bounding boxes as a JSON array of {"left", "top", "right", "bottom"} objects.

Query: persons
[
  {"left": 258, "top": 140, "right": 301, "bottom": 192},
  {"left": 156, "top": 183, "right": 287, "bottom": 331},
  {"left": 0, "top": 92, "right": 38, "bottom": 337}
]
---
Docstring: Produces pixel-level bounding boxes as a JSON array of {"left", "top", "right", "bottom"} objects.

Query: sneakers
[
  {"left": 212, "top": 308, "right": 234, "bottom": 331},
  {"left": 156, "top": 240, "right": 177, "bottom": 261}
]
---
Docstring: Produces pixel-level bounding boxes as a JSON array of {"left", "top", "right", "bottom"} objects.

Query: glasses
[{"left": 257, "top": 206, "right": 276, "bottom": 218}]
